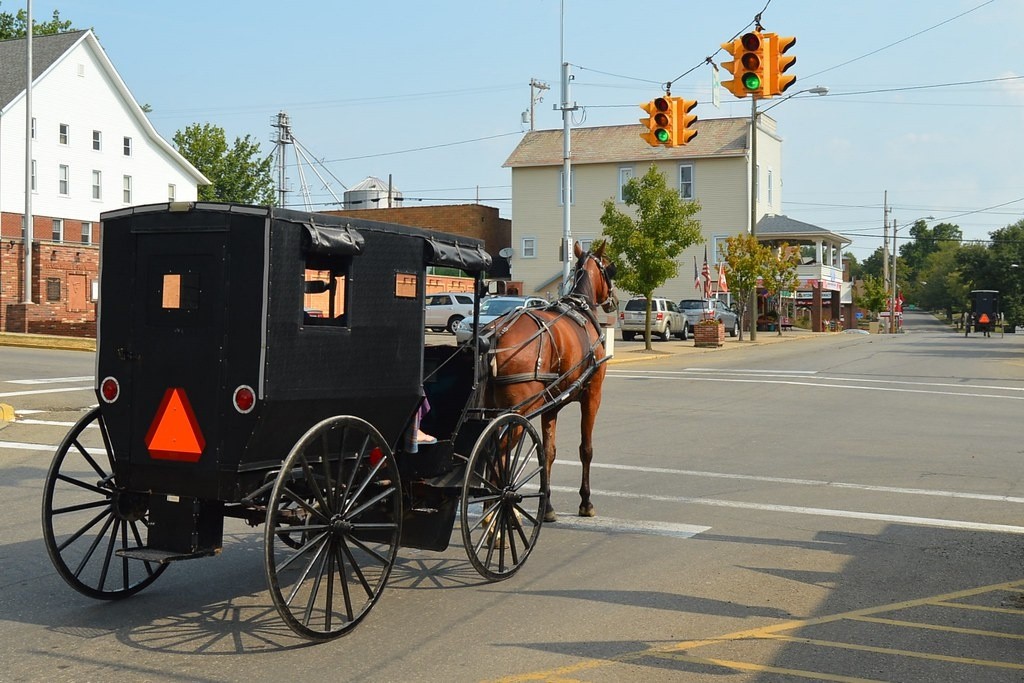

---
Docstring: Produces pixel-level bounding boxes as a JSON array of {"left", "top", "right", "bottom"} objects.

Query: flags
[{"left": 694, "top": 245, "right": 727, "bottom": 299}]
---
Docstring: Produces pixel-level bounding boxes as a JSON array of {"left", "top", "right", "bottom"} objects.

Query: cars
[{"left": 455, "top": 297, "right": 552, "bottom": 349}]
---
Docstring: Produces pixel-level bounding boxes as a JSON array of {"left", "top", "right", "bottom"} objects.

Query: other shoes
[{"left": 417, "top": 429, "right": 437, "bottom": 444}]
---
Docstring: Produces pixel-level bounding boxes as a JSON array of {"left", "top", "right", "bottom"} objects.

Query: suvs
[
  {"left": 676, "top": 298, "right": 740, "bottom": 337},
  {"left": 621, "top": 296, "right": 689, "bottom": 342},
  {"left": 422, "top": 293, "right": 482, "bottom": 335}
]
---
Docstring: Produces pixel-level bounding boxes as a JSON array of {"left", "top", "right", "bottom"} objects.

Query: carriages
[
  {"left": 962, "top": 289, "right": 1003, "bottom": 339},
  {"left": 41, "top": 200, "right": 620, "bottom": 643}
]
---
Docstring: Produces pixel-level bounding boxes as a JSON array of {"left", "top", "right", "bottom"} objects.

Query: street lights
[
  {"left": 890, "top": 216, "right": 935, "bottom": 335},
  {"left": 750, "top": 86, "right": 830, "bottom": 342},
  {"left": 1011, "top": 264, "right": 1023, "bottom": 269}
]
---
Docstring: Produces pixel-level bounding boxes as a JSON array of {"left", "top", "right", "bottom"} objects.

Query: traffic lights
[
  {"left": 720, "top": 25, "right": 798, "bottom": 99},
  {"left": 639, "top": 96, "right": 699, "bottom": 149}
]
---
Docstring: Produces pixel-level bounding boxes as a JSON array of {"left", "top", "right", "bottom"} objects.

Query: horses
[{"left": 477, "top": 238, "right": 620, "bottom": 550}]
